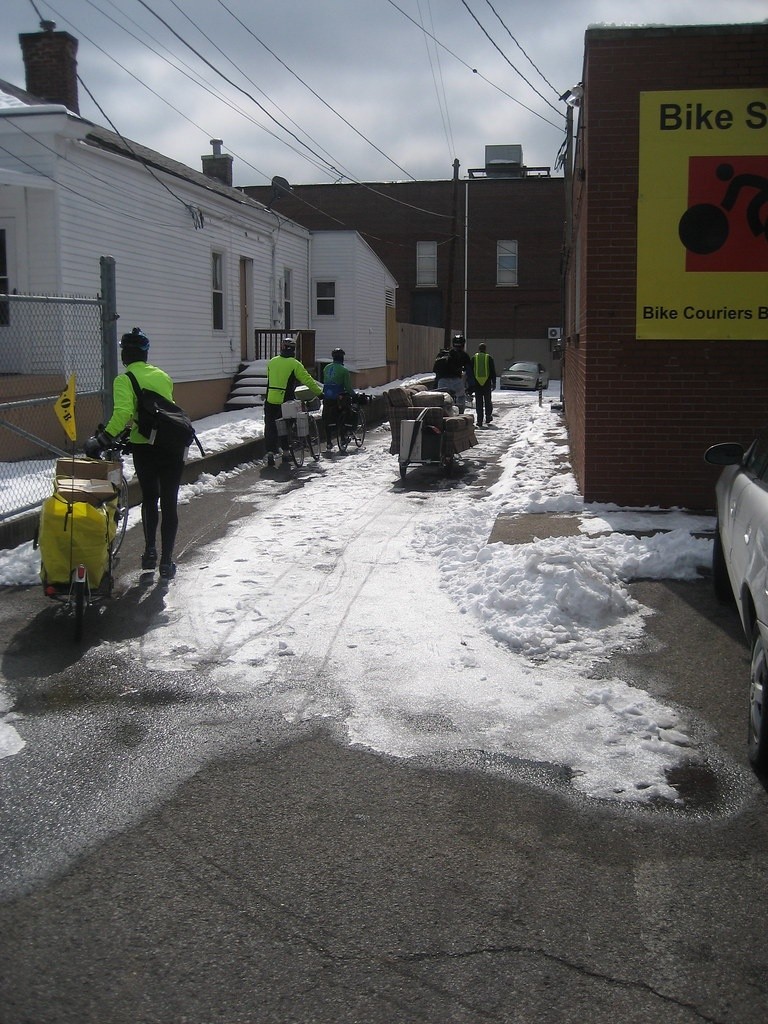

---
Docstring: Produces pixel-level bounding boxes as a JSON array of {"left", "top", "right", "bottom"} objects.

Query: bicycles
[
  {"left": 66, "top": 442, "right": 128, "bottom": 626},
  {"left": 289, "top": 395, "right": 321, "bottom": 469},
  {"left": 336, "top": 393, "right": 373, "bottom": 452}
]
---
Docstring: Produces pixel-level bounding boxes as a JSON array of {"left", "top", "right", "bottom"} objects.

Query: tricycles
[{"left": 399, "top": 389, "right": 469, "bottom": 477}]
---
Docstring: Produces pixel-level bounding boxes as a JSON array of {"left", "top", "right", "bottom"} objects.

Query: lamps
[{"left": 569, "top": 81, "right": 583, "bottom": 108}]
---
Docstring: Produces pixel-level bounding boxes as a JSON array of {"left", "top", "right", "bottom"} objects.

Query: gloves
[
  {"left": 318, "top": 391, "right": 326, "bottom": 400},
  {"left": 84, "top": 429, "right": 116, "bottom": 460}
]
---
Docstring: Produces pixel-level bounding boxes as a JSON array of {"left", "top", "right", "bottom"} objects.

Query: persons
[
  {"left": 433, "top": 333, "right": 471, "bottom": 414},
  {"left": 469, "top": 343, "right": 496, "bottom": 427},
  {"left": 84, "top": 326, "right": 188, "bottom": 580},
  {"left": 322, "top": 347, "right": 357, "bottom": 451},
  {"left": 265, "top": 338, "right": 324, "bottom": 466}
]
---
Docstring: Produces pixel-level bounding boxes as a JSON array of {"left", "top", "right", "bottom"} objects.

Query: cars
[
  {"left": 500, "top": 362, "right": 549, "bottom": 390},
  {"left": 705, "top": 434, "right": 768, "bottom": 770}
]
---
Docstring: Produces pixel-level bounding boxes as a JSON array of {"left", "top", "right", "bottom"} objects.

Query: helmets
[
  {"left": 118, "top": 327, "right": 150, "bottom": 351},
  {"left": 331, "top": 347, "right": 346, "bottom": 357},
  {"left": 282, "top": 338, "right": 296, "bottom": 350},
  {"left": 452, "top": 334, "right": 466, "bottom": 346}
]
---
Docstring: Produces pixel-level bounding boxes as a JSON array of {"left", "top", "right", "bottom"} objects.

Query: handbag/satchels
[{"left": 136, "top": 387, "right": 194, "bottom": 452}]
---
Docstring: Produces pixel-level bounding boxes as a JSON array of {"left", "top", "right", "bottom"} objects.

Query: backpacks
[{"left": 432, "top": 346, "right": 459, "bottom": 375}]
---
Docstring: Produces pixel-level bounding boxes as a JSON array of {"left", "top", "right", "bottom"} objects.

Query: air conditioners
[{"left": 548, "top": 328, "right": 563, "bottom": 339}]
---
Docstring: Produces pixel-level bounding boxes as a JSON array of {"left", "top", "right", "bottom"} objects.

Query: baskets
[{"left": 301, "top": 397, "right": 321, "bottom": 413}]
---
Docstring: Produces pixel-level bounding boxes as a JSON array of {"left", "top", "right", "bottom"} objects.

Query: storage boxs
[
  {"left": 56, "top": 457, "right": 123, "bottom": 487},
  {"left": 281, "top": 399, "right": 302, "bottom": 419},
  {"left": 56, "top": 477, "right": 116, "bottom": 508}
]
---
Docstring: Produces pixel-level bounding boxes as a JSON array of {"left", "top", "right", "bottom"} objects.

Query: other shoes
[
  {"left": 327, "top": 443, "right": 335, "bottom": 450},
  {"left": 140, "top": 547, "right": 157, "bottom": 573},
  {"left": 267, "top": 452, "right": 276, "bottom": 466},
  {"left": 159, "top": 561, "right": 176, "bottom": 581},
  {"left": 282, "top": 453, "right": 295, "bottom": 462},
  {"left": 486, "top": 416, "right": 493, "bottom": 423},
  {"left": 476, "top": 421, "right": 482, "bottom": 427},
  {"left": 340, "top": 436, "right": 351, "bottom": 446}
]
